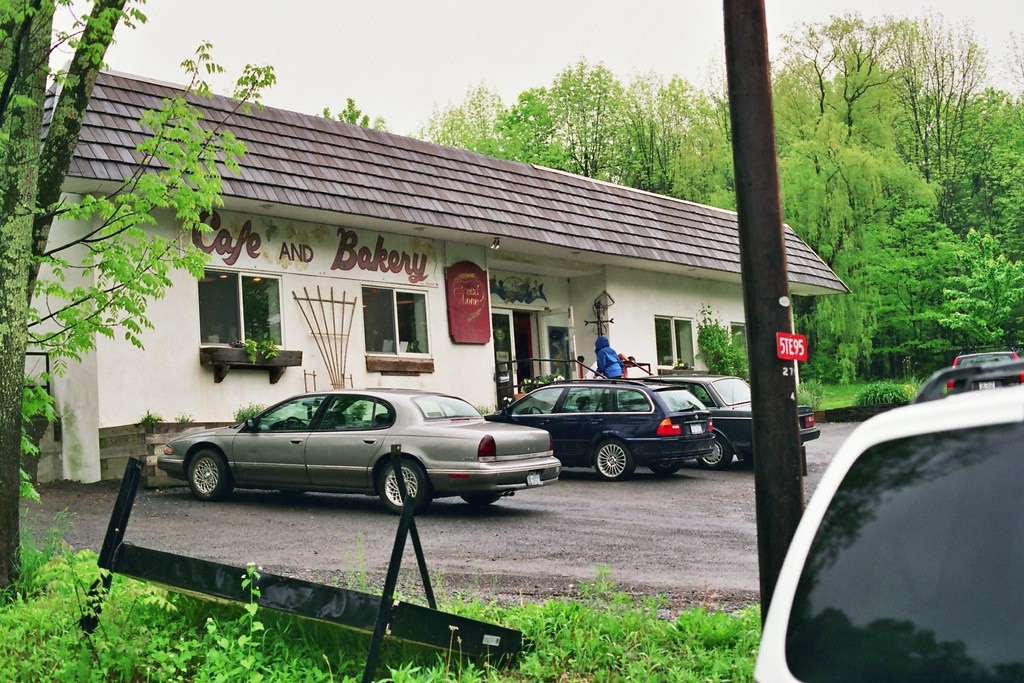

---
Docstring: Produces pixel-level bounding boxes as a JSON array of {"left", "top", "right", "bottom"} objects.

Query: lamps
[{"left": 491, "top": 236, "right": 499, "bottom": 249}]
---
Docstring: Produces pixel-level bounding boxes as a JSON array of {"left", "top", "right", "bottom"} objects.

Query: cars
[
  {"left": 484, "top": 375, "right": 717, "bottom": 482},
  {"left": 156, "top": 388, "right": 562, "bottom": 511},
  {"left": 903, "top": 352, "right": 1024, "bottom": 405},
  {"left": 637, "top": 375, "right": 821, "bottom": 471}
]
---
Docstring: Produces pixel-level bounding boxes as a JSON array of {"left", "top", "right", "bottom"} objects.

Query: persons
[{"left": 593, "top": 336, "right": 625, "bottom": 378}]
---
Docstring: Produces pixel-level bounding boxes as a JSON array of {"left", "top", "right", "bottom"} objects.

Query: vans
[{"left": 755, "top": 388, "right": 1024, "bottom": 683}]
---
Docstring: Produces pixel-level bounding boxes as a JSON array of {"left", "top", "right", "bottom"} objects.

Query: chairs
[
  {"left": 374, "top": 413, "right": 390, "bottom": 427},
  {"left": 576, "top": 397, "right": 590, "bottom": 413},
  {"left": 318, "top": 411, "right": 346, "bottom": 430}
]
